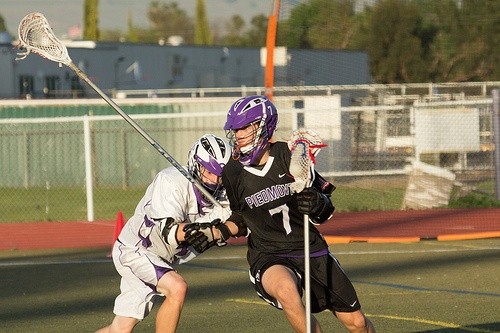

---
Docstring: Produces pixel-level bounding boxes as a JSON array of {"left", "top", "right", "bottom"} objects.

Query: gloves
[
  {"left": 296, "top": 187, "right": 328, "bottom": 220},
  {"left": 183, "top": 218, "right": 230, "bottom": 253}
]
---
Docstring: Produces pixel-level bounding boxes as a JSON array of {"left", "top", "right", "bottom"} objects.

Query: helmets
[
  {"left": 224, "top": 95, "right": 278, "bottom": 165},
  {"left": 187, "top": 134, "right": 233, "bottom": 181}
]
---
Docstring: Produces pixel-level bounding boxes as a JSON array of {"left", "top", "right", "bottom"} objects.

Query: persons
[
  {"left": 181, "top": 97, "right": 379, "bottom": 333},
  {"left": 89, "top": 132, "right": 235, "bottom": 333}
]
